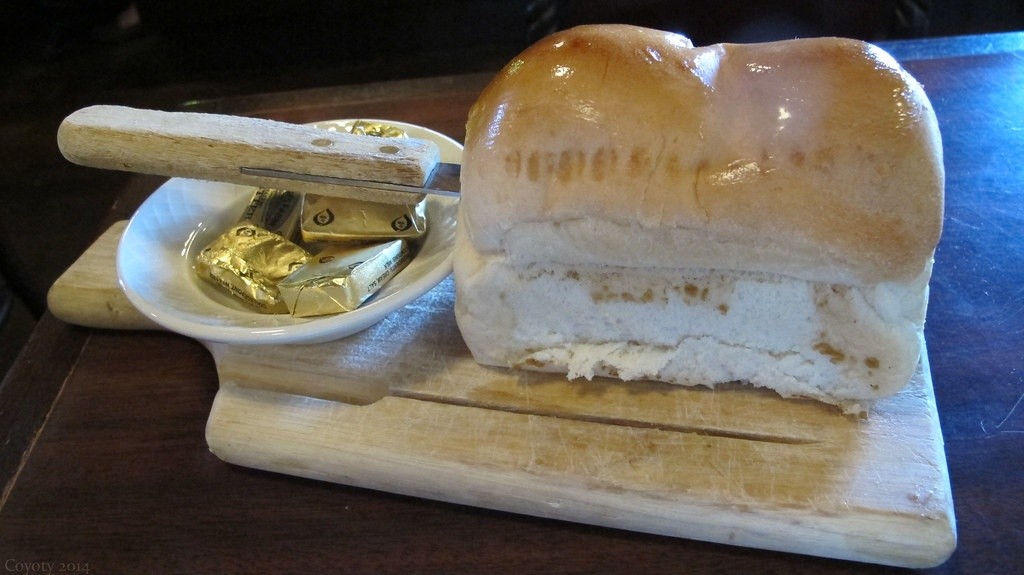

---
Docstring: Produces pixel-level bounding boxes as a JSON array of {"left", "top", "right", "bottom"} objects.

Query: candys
[{"left": 191, "top": 113, "right": 427, "bottom": 318}]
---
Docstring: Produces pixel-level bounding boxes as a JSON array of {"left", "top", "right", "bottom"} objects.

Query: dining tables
[{"left": 1, "top": 34, "right": 1024, "bottom": 575}]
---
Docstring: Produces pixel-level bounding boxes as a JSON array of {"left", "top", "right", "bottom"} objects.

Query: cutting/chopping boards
[{"left": 43, "top": 220, "right": 959, "bottom": 571}]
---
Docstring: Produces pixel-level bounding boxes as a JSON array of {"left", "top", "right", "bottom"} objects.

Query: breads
[{"left": 453, "top": 25, "right": 946, "bottom": 412}]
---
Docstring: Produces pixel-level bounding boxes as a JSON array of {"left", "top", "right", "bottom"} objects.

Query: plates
[{"left": 116, "top": 117, "right": 468, "bottom": 350}]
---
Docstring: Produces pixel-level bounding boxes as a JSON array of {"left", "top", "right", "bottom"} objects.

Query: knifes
[{"left": 54, "top": 103, "right": 462, "bottom": 211}]
